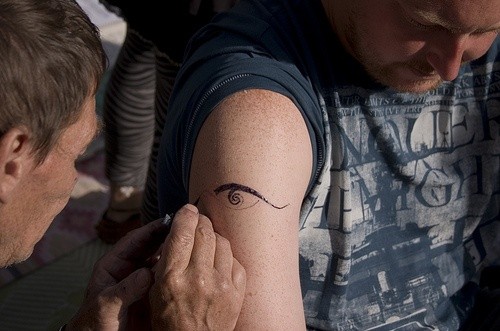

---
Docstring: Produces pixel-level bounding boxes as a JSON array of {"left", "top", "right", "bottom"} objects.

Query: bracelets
[{"left": 59, "top": 324, "right": 66, "bottom": 331}]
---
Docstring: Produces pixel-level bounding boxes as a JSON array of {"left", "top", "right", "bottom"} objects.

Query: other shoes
[{"left": 96, "top": 209, "right": 141, "bottom": 244}]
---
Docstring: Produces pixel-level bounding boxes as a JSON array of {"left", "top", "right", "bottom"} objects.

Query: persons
[
  {"left": 154, "top": 1, "right": 500, "bottom": 330},
  {"left": 0, "top": 1, "right": 247, "bottom": 331},
  {"left": 95, "top": 0, "right": 201, "bottom": 263}
]
